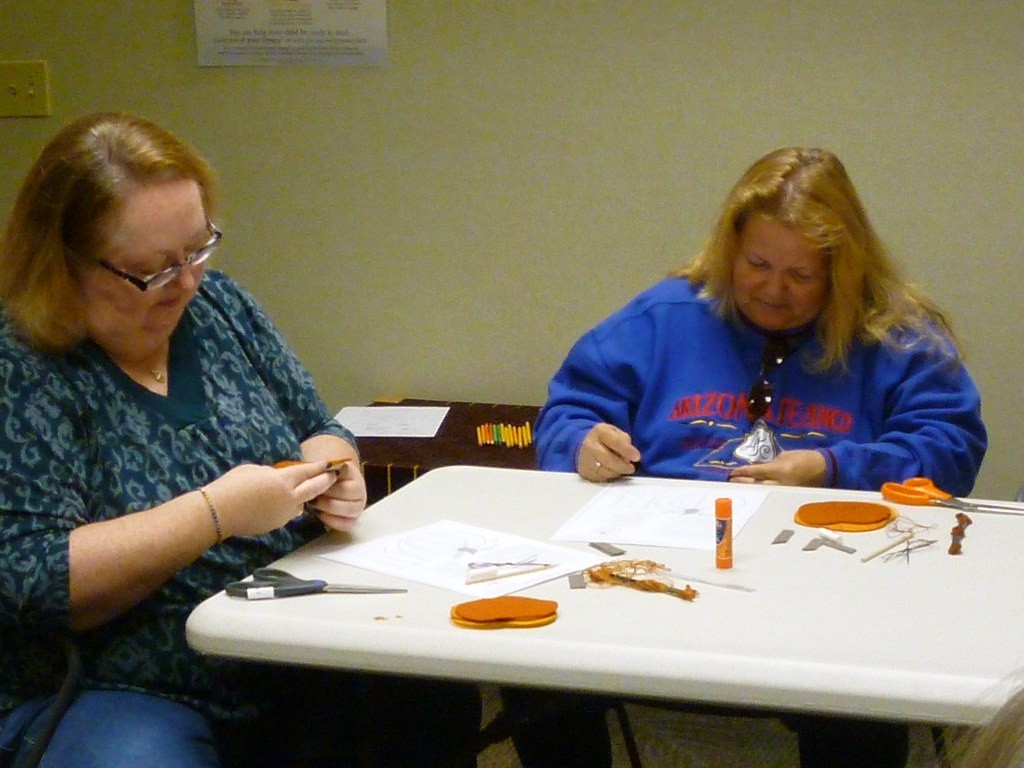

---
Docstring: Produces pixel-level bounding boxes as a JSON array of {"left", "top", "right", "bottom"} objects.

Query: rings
[
  {"left": 595, "top": 462, "right": 601, "bottom": 471},
  {"left": 297, "top": 503, "right": 303, "bottom": 516}
]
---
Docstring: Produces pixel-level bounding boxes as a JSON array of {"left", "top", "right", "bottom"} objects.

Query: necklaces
[{"left": 117, "top": 356, "right": 164, "bottom": 383}]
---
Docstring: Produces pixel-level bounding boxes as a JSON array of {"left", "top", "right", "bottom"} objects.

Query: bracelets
[{"left": 201, "top": 487, "right": 222, "bottom": 545}]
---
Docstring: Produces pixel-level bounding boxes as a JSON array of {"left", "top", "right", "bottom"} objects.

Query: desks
[
  {"left": 355, "top": 397, "right": 539, "bottom": 505},
  {"left": 190, "top": 462, "right": 1024, "bottom": 768}
]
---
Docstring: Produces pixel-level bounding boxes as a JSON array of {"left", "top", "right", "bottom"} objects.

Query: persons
[
  {"left": 498, "top": 146, "right": 988, "bottom": 768},
  {"left": 0, "top": 113, "right": 483, "bottom": 768}
]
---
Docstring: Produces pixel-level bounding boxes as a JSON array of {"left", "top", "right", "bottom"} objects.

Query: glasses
[
  {"left": 748, "top": 336, "right": 786, "bottom": 418},
  {"left": 81, "top": 217, "right": 223, "bottom": 291}
]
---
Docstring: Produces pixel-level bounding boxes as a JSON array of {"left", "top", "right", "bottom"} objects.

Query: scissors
[
  {"left": 882, "top": 477, "right": 1024, "bottom": 517},
  {"left": 225, "top": 568, "right": 407, "bottom": 601}
]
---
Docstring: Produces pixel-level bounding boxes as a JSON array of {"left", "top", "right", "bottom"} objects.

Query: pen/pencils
[{"left": 476, "top": 421, "right": 531, "bottom": 448}]
